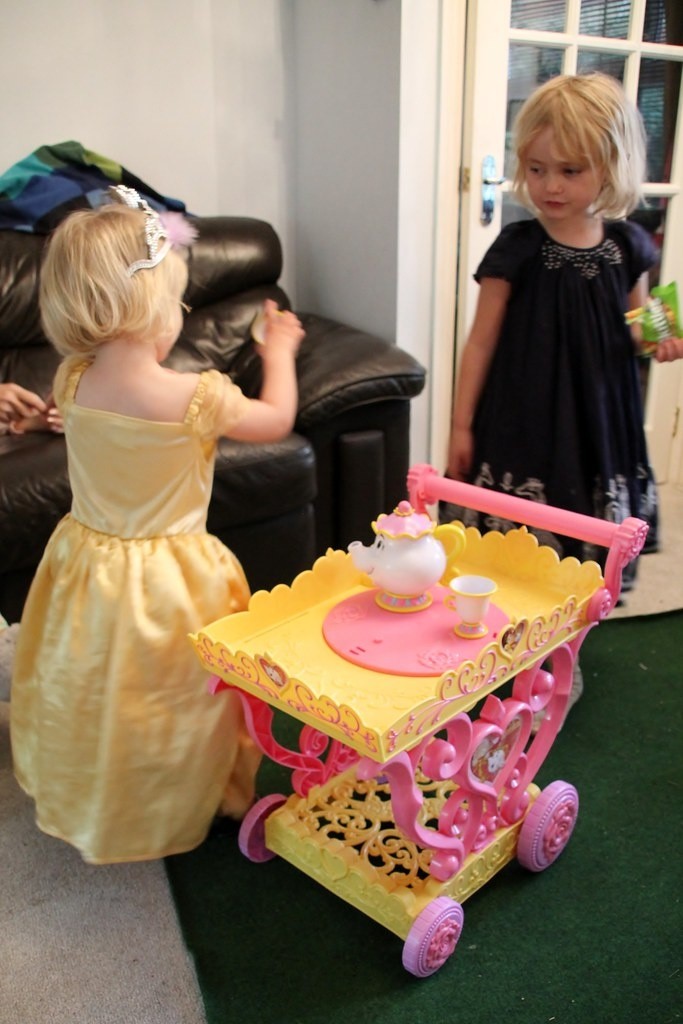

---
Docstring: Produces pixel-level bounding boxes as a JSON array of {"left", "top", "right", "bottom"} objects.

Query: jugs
[{"left": 347, "top": 499, "right": 465, "bottom": 613}]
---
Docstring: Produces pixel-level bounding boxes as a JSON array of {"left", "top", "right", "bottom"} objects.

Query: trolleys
[{"left": 183, "top": 465, "right": 651, "bottom": 980}]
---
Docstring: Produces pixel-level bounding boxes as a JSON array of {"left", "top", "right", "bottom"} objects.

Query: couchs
[{"left": 0, "top": 212, "right": 426, "bottom": 626}]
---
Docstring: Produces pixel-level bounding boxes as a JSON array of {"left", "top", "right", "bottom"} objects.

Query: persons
[
  {"left": 437, "top": 68, "right": 683, "bottom": 648},
  {"left": 0, "top": 185, "right": 305, "bottom": 867}
]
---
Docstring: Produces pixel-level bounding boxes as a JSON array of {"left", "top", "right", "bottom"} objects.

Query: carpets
[{"left": 163, "top": 604, "right": 683, "bottom": 1024}]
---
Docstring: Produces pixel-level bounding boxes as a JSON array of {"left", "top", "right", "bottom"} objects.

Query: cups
[{"left": 450, "top": 575, "right": 498, "bottom": 639}]
